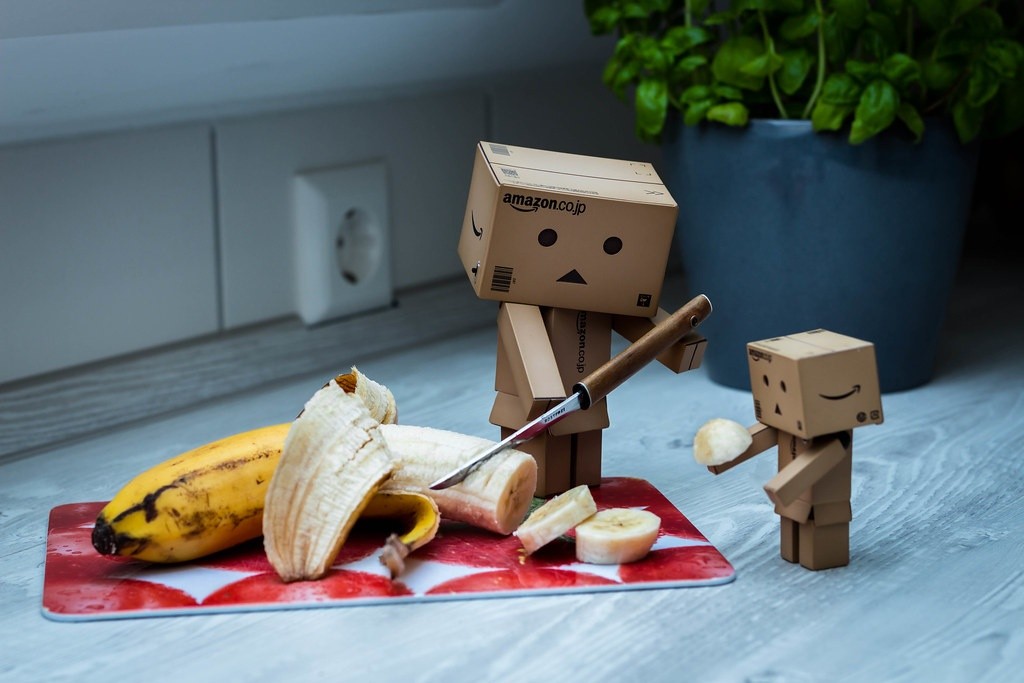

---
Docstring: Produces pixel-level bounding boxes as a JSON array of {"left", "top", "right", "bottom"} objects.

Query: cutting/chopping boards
[{"left": 42, "top": 476, "right": 736, "bottom": 622}]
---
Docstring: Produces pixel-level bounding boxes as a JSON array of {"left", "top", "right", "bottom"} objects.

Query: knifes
[{"left": 430, "top": 294, "right": 714, "bottom": 490}]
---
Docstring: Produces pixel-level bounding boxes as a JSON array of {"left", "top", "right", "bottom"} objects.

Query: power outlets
[{"left": 289, "top": 158, "right": 394, "bottom": 327}]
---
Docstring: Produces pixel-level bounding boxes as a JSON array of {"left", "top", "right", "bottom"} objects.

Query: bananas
[
  {"left": 692, "top": 416, "right": 751, "bottom": 466},
  {"left": 92, "top": 364, "right": 660, "bottom": 592}
]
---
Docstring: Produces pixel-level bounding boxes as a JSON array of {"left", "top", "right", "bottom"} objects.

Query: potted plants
[{"left": 580, "top": 1, "right": 1024, "bottom": 396}]
